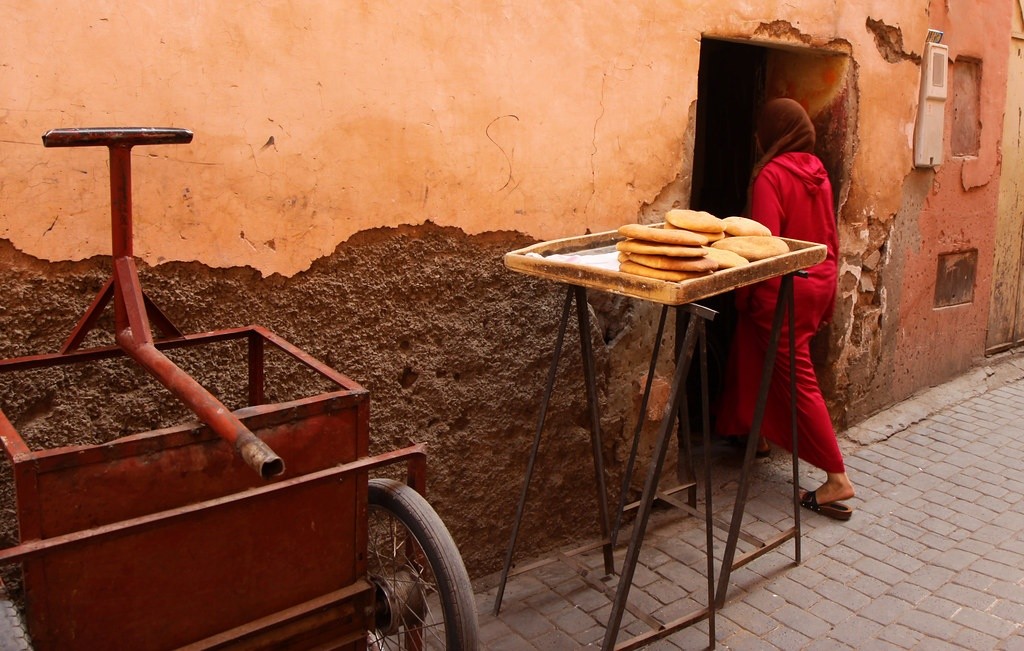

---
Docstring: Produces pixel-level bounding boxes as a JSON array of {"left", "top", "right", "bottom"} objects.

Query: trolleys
[{"left": 0, "top": 128, "right": 482, "bottom": 651}]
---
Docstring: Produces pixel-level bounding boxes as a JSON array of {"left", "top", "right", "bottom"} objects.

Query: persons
[{"left": 718, "top": 99, "right": 855, "bottom": 520}]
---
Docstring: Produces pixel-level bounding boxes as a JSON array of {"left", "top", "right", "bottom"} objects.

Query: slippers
[
  {"left": 756, "top": 447, "right": 773, "bottom": 458},
  {"left": 799, "top": 491, "right": 853, "bottom": 520}
]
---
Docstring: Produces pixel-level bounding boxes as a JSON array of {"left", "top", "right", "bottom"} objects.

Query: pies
[{"left": 615, "top": 209, "right": 790, "bottom": 284}]
